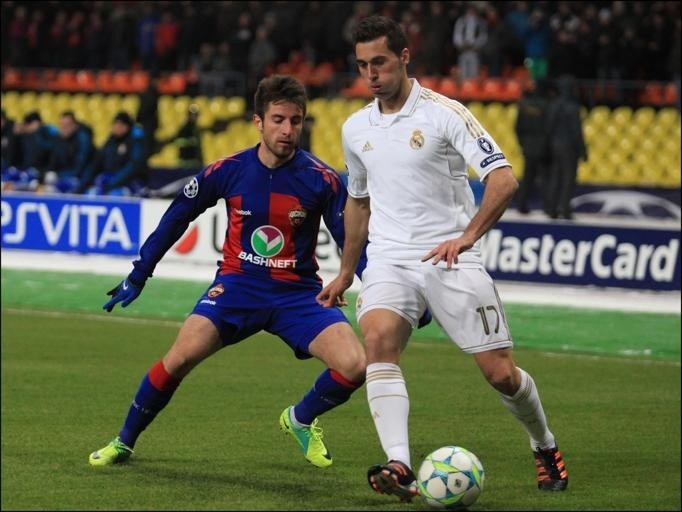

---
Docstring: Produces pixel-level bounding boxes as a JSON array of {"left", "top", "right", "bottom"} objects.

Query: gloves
[
  {"left": 418, "top": 309, "right": 432, "bottom": 329},
  {"left": 103, "top": 278, "right": 140, "bottom": 312}
]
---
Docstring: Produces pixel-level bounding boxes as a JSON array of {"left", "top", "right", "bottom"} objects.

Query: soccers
[{"left": 417, "top": 445, "right": 484, "bottom": 509}]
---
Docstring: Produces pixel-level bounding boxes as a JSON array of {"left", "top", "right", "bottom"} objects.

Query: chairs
[
  {"left": 2, "top": 65, "right": 248, "bottom": 171},
  {"left": 468, "top": 100, "right": 680, "bottom": 188}
]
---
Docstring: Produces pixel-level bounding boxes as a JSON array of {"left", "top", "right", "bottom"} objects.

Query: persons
[
  {"left": 86, "top": 73, "right": 433, "bottom": 469},
  {"left": 314, "top": 14, "right": 569, "bottom": 500},
  {"left": 0, "top": 106, "right": 204, "bottom": 195},
  {"left": 513, "top": 75, "right": 588, "bottom": 220}
]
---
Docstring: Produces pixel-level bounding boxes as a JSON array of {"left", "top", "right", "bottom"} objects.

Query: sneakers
[
  {"left": 533, "top": 447, "right": 568, "bottom": 492},
  {"left": 368, "top": 459, "right": 421, "bottom": 504},
  {"left": 279, "top": 405, "right": 333, "bottom": 468},
  {"left": 88, "top": 436, "right": 133, "bottom": 466}
]
